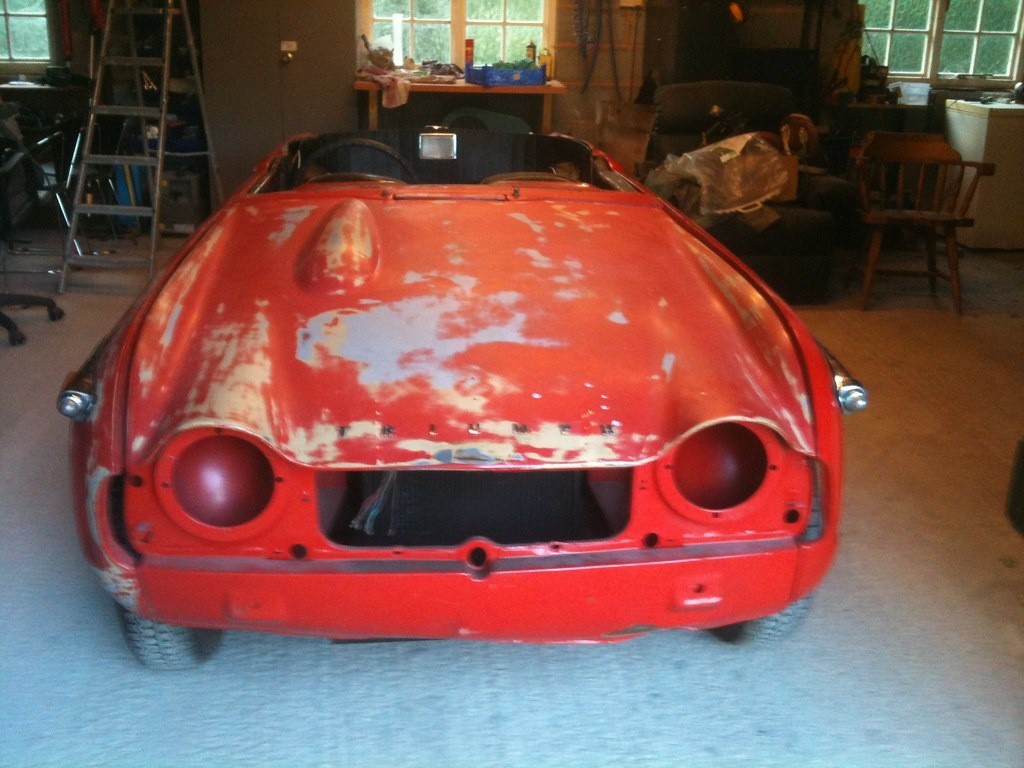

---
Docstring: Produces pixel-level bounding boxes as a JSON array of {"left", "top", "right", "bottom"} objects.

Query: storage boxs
[
  {"left": 465, "top": 62, "right": 547, "bottom": 87},
  {"left": 898, "top": 82, "right": 931, "bottom": 106}
]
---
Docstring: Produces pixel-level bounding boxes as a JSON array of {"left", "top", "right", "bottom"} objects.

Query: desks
[{"left": 353, "top": 78, "right": 568, "bottom": 134}]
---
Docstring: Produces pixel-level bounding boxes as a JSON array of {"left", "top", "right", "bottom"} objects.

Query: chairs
[
  {"left": 44, "top": 114, "right": 138, "bottom": 249},
  {"left": 853, "top": 132, "right": 995, "bottom": 314},
  {"left": 0, "top": 131, "right": 66, "bottom": 347}
]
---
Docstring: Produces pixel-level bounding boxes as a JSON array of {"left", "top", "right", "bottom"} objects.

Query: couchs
[{"left": 650, "top": 81, "right": 860, "bottom": 305}]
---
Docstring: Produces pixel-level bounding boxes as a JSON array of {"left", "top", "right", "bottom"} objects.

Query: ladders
[{"left": 57, "top": 0, "right": 225, "bottom": 296}]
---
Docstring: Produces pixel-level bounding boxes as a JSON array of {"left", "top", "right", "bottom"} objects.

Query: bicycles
[
  {"left": 53, "top": 132, "right": 872, "bottom": 670},
  {"left": 0, "top": 114, "right": 120, "bottom": 234}
]
